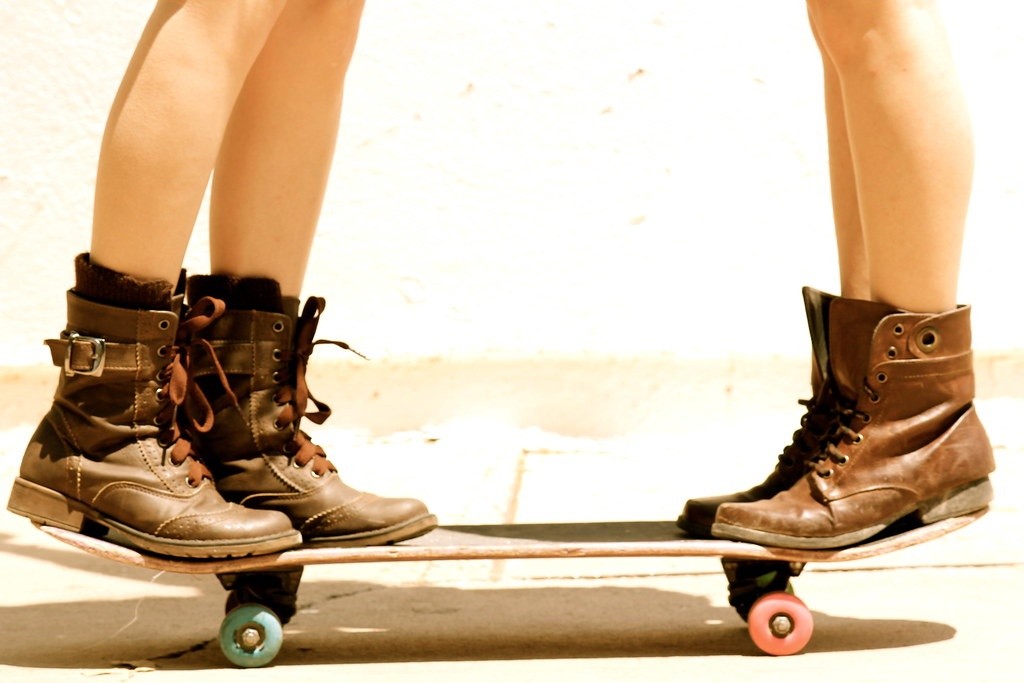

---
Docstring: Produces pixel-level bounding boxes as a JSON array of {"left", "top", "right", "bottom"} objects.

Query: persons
[
  {"left": 0, "top": 0, "right": 437, "bottom": 560},
  {"left": 675, "top": 0, "right": 998, "bottom": 551}
]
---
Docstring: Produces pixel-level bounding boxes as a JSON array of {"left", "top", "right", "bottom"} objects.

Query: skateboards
[{"left": 29, "top": 502, "right": 993, "bottom": 668}]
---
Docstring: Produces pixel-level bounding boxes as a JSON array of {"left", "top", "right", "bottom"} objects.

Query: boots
[
  {"left": 710, "top": 296, "right": 997, "bottom": 549},
  {"left": 182, "top": 273, "right": 439, "bottom": 550},
  {"left": 5, "top": 253, "right": 303, "bottom": 558},
  {"left": 676, "top": 285, "right": 837, "bottom": 538}
]
